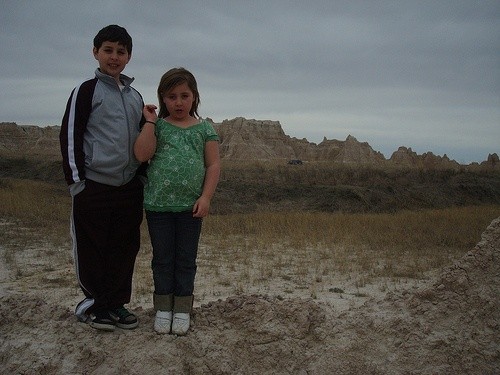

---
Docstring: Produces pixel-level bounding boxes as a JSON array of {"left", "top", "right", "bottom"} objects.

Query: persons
[
  {"left": 59, "top": 24, "right": 145, "bottom": 332},
  {"left": 133, "top": 68, "right": 222, "bottom": 335}
]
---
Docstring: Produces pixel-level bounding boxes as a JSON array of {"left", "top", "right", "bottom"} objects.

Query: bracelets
[{"left": 145, "top": 120, "right": 157, "bottom": 126}]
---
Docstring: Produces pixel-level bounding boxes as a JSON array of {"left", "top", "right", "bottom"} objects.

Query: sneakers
[
  {"left": 153, "top": 309, "right": 172, "bottom": 334},
  {"left": 86, "top": 312, "right": 115, "bottom": 332},
  {"left": 171, "top": 311, "right": 190, "bottom": 335},
  {"left": 110, "top": 305, "right": 140, "bottom": 329}
]
